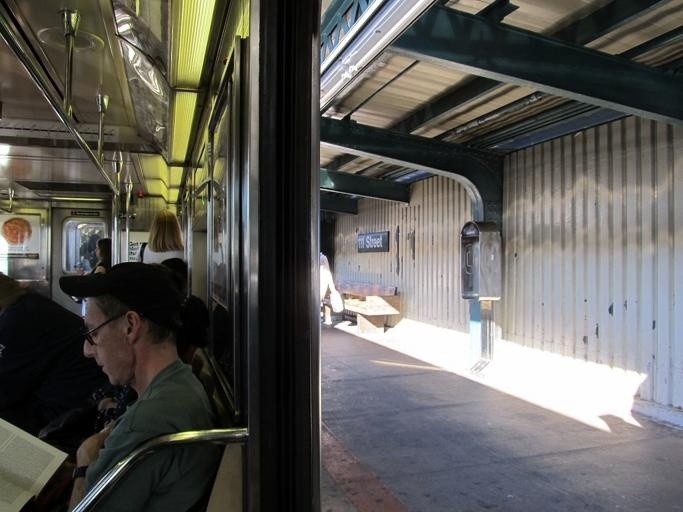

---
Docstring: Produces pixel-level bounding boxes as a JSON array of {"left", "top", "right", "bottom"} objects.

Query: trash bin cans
[{"left": 72, "top": 465, "right": 88, "bottom": 479}]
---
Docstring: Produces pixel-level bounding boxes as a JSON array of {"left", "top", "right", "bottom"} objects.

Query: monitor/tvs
[{"left": 0, "top": 418, "right": 69, "bottom": 512}]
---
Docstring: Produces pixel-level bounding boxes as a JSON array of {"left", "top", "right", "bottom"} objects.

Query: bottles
[{"left": 58, "top": 261, "right": 183, "bottom": 332}]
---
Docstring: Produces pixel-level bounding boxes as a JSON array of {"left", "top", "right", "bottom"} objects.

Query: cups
[{"left": 79, "top": 311, "right": 127, "bottom": 345}]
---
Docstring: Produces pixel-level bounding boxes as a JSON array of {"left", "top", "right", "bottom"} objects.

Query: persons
[
  {"left": 88, "top": 238, "right": 112, "bottom": 274},
  {"left": 80, "top": 242, "right": 94, "bottom": 272},
  {"left": 57, "top": 258, "right": 220, "bottom": 512},
  {"left": 136, "top": 208, "right": 185, "bottom": 264},
  {"left": 0, "top": 271, "right": 103, "bottom": 437}
]
